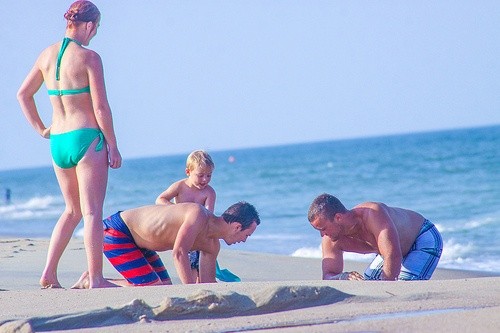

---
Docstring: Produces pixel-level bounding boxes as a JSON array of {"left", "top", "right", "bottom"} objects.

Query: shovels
[{"left": 215, "top": 259, "right": 242, "bottom": 283}]
